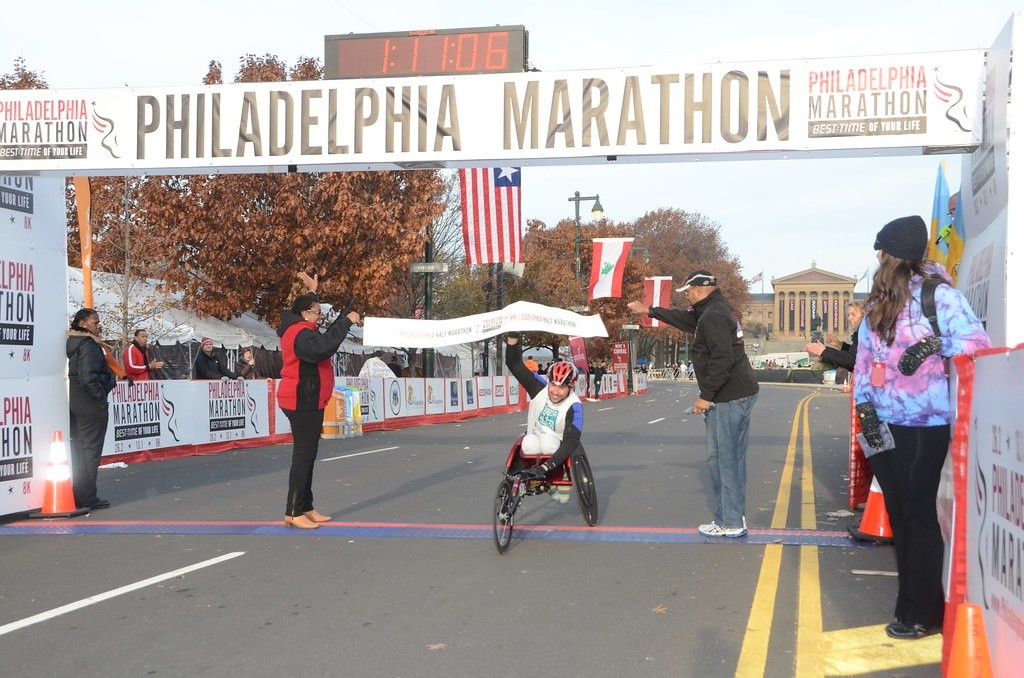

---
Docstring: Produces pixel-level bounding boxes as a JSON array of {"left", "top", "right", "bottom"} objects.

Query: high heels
[
  {"left": 303, "top": 509, "right": 331, "bottom": 522},
  {"left": 285, "top": 515, "right": 319, "bottom": 529}
]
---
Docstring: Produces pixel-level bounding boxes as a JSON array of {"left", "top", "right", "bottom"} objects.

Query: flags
[
  {"left": 752, "top": 272, "right": 761, "bottom": 282},
  {"left": 460, "top": 168, "right": 523, "bottom": 265},
  {"left": 643, "top": 276, "right": 672, "bottom": 327},
  {"left": 589, "top": 238, "right": 634, "bottom": 300}
]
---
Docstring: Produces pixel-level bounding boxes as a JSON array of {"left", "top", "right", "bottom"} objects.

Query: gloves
[
  {"left": 509, "top": 331, "right": 520, "bottom": 337},
  {"left": 898, "top": 336, "right": 942, "bottom": 376},
  {"left": 530, "top": 463, "right": 549, "bottom": 475},
  {"left": 597, "top": 381, "right": 600, "bottom": 385},
  {"left": 855, "top": 401, "right": 883, "bottom": 448}
]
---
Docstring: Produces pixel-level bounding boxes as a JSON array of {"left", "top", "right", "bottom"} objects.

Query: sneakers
[
  {"left": 887, "top": 617, "right": 943, "bottom": 637},
  {"left": 698, "top": 516, "right": 748, "bottom": 537}
]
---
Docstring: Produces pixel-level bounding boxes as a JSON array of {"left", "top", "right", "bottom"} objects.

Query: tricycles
[{"left": 491, "top": 431, "right": 598, "bottom": 555}]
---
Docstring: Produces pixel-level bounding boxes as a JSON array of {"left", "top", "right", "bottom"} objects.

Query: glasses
[{"left": 308, "top": 309, "right": 321, "bottom": 315}]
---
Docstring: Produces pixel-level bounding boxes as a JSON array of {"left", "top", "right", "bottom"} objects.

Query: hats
[
  {"left": 201, "top": 337, "right": 213, "bottom": 346},
  {"left": 675, "top": 270, "right": 716, "bottom": 291},
  {"left": 391, "top": 356, "right": 396, "bottom": 360},
  {"left": 874, "top": 215, "right": 928, "bottom": 260},
  {"left": 538, "top": 364, "right": 542, "bottom": 366}
]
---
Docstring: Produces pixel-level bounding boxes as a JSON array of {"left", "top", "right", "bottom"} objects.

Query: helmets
[{"left": 547, "top": 361, "right": 579, "bottom": 387}]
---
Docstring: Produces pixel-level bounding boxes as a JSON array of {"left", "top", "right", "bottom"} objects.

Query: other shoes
[
  {"left": 79, "top": 500, "right": 110, "bottom": 507},
  {"left": 856, "top": 503, "right": 866, "bottom": 510}
]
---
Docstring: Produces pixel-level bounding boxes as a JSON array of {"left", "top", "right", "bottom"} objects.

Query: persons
[
  {"left": 505, "top": 331, "right": 584, "bottom": 475},
  {"left": 65, "top": 308, "right": 134, "bottom": 509},
  {"left": 673, "top": 360, "right": 693, "bottom": 380},
  {"left": 375, "top": 350, "right": 402, "bottom": 377},
  {"left": 588, "top": 361, "right": 608, "bottom": 400},
  {"left": 277, "top": 272, "right": 360, "bottom": 530},
  {"left": 236, "top": 347, "right": 255, "bottom": 379},
  {"left": 123, "top": 330, "right": 163, "bottom": 380},
  {"left": 766, "top": 359, "right": 790, "bottom": 368},
  {"left": 638, "top": 365, "right": 647, "bottom": 373},
  {"left": 628, "top": 270, "right": 759, "bottom": 538},
  {"left": 195, "top": 337, "right": 244, "bottom": 383},
  {"left": 525, "top": 356, "right": 562, "bottom": 375},
  {"left": 807, "top": 301, "right": 862, "bottom": 372},
  {"left": 856, "top": 217, "right": 990, "bottom": 639}
]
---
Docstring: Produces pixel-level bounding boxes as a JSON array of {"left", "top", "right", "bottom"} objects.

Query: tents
[
  {"left": 637, "top": 359, "right": 649, "bottom": 364},
  {"left": 67, "top": 266, "right": 609, "bottom": 381}
]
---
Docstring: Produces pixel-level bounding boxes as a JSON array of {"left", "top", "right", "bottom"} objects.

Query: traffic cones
[
  {"left": 946, "top": 603, "right": 995, "bottom": 678},
  {"left": 29, "top": 430, "right": 91, "bottom": 519},
  {"left": 847, "top": 473, "right": 894, "bottom": 544}
]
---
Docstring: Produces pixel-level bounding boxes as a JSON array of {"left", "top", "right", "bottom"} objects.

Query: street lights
[{"left": 568, "top": 191, "right": 604, "bottom": 282}]
